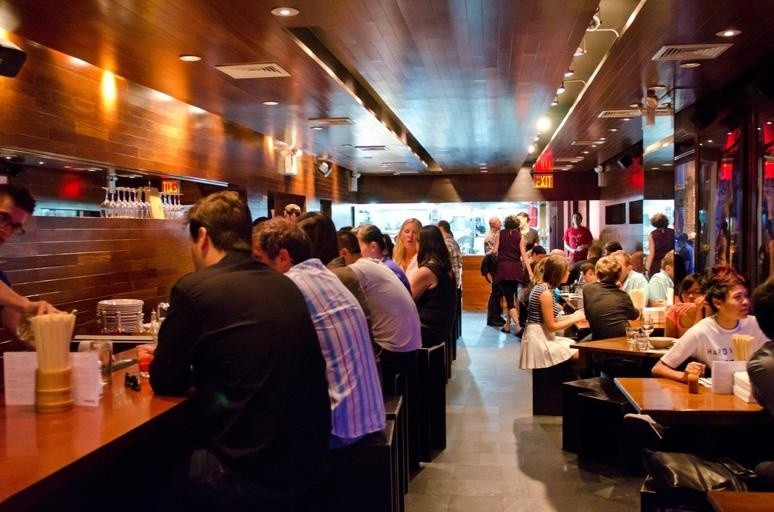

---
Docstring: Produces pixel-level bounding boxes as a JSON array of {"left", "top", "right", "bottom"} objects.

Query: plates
[{"left": 95, "top": 298, "right": 145, "bottom": 332}]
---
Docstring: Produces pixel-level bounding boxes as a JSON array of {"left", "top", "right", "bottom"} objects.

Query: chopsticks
[
  {"left": 30, "top": 312, "right": 77, "bottom": 370},
  {"left": 731, "top": 334, "right": 755, "bottom": 362}
]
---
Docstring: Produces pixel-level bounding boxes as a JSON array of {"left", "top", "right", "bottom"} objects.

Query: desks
[{"left": 0, "top": 343, "right": 201, "bottom": 507}]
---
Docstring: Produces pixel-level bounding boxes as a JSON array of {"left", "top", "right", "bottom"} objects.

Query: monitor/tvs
[
  {"left": 629, "top": 200, "right": 643, "bottom": 224},
  {"left": 605, "top": 203, "right": 626, "bottom": 224}
]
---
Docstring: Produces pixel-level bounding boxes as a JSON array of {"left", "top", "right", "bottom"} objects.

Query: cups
[
  {"left": 688, "top": 372, "right": 699, "bottom": 394},
  {"left": 136, "top": 343, "right": 158, "bottom": 378},
  {"left": 88, "top": 341, "right": 114, "bottom": 386},
  {"left": 633, "top": 333, "right": 649, "bottom": 350},
  {"left": 15, "top": 314, "right": 37, "bottom": 344},
  {"left": 33, "top": 369, "right": 73, "bottom": 414},
  {"left": 625, "top": 326, "right": 640, "bottom": 345}
]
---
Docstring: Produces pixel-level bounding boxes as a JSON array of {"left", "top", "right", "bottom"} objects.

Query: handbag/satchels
[{"left": 637, "top": 446, "right": 758, "bottom": 497}]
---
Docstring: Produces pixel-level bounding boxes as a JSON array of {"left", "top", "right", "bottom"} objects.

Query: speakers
[
  {"left": 617, "top": 154, "right": 633, "bottom": 169},
  {"left": 0, "top": 41, "right": 26, "bottom": 78},
  {"left": 317, "top": 162, "right": 333, "bottom": 177}
]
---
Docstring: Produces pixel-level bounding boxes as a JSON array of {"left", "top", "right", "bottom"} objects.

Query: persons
[
  {"left": 147, "top": 186, "right": 463, "bottom": 497},
  {"left": 519, "top": 242, "right": 774, "bottom": 481},
  {"left": 644, "top": 213, "right": 675, "bottom": 278},
  {"left": 562, "top": 213, "right": 594, "bottom": 261},
  {"left": 482, "top": 212, "right": 539, "bottom": 327},
  {"left": 0, "top": 183, "right": 61, "bottom": 347}
]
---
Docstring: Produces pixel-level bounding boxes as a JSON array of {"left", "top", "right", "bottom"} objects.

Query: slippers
[
  {"left": 499, "top": 327, "right": 511, "bottom": 333},
  {"left": 514, "top": 327, "right": 523, "bottom": 336}
]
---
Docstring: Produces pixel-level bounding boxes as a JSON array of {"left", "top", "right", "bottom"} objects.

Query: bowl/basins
[{"left": 646, "top": 338, "right": 673, "bottom": 349}]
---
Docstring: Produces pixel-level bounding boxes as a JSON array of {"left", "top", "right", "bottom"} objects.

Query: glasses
[{"left": 0, "top": 213, "right": 26, "bottom": 237}]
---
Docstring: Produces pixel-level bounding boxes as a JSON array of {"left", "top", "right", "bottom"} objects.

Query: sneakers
[{"left": 487, "top": 317, "right": 506, "bottom": 326}]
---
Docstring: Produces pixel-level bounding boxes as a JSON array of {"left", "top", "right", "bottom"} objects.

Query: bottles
[{"left": 96, "top": 310, "right": 159, "bottom": 335}]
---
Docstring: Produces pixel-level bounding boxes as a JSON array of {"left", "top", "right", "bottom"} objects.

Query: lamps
[
  {"left": 313, "top": 155, "right": 333, "bottom": 178},
  {"left": 618, "top": 151, "right": 642, "bottom": 170}
]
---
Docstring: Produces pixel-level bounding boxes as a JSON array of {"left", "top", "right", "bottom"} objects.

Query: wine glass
[
  {"left": 156, "top": 191, "right": 184, "bottom": 218},
  {"left": 98, "top": 185, "right": 151, "bottom": 219},
  {"left": 639, "top": 314, "right": 655, "bottom": 347}
]
